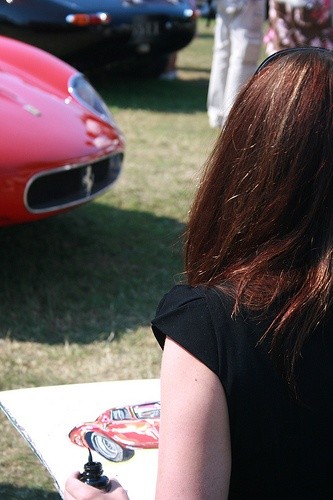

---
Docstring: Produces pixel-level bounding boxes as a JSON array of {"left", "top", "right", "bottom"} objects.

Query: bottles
[{"left": 78, "top": 462, "right": 112, "bottom": 494}]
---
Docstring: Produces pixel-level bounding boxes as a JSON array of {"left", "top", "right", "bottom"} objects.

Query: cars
[{"left": 0, "top": 0, "right": 197, "bottom": 80}]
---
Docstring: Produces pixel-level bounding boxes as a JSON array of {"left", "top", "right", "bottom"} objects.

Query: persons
[
  {"left": 204, "top": 1, "right": 270, "bottom": 127},
  {"left": 62, "top": 45, "right": 333, "bottom": 500},
  {"left": 264, "top": 0, "right": 333, "bottom": 54}
]
